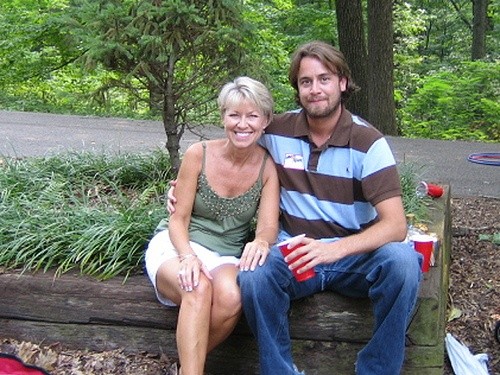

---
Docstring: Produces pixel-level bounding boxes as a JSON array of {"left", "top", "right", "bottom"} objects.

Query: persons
[
  {"left": 166, "top": 42, "right": 424, "bottom": 375},
  {"left": 144, "top": 76, "right": 280, "bottom": 375}
]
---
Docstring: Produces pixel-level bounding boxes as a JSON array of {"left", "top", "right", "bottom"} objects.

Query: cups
[
  {"left": 276, "top": 234, "right": 315, "bottom": 282},
  {"left": 416, "top": 181, "right": 443, "bottom": 198},
  {"left": 410, "top": 235, "right": 433, "bottom": 271}
]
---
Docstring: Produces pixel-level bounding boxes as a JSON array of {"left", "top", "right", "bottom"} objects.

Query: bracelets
[{"left": 180, "top": 254, "right": 197, "bottom": 263}]
